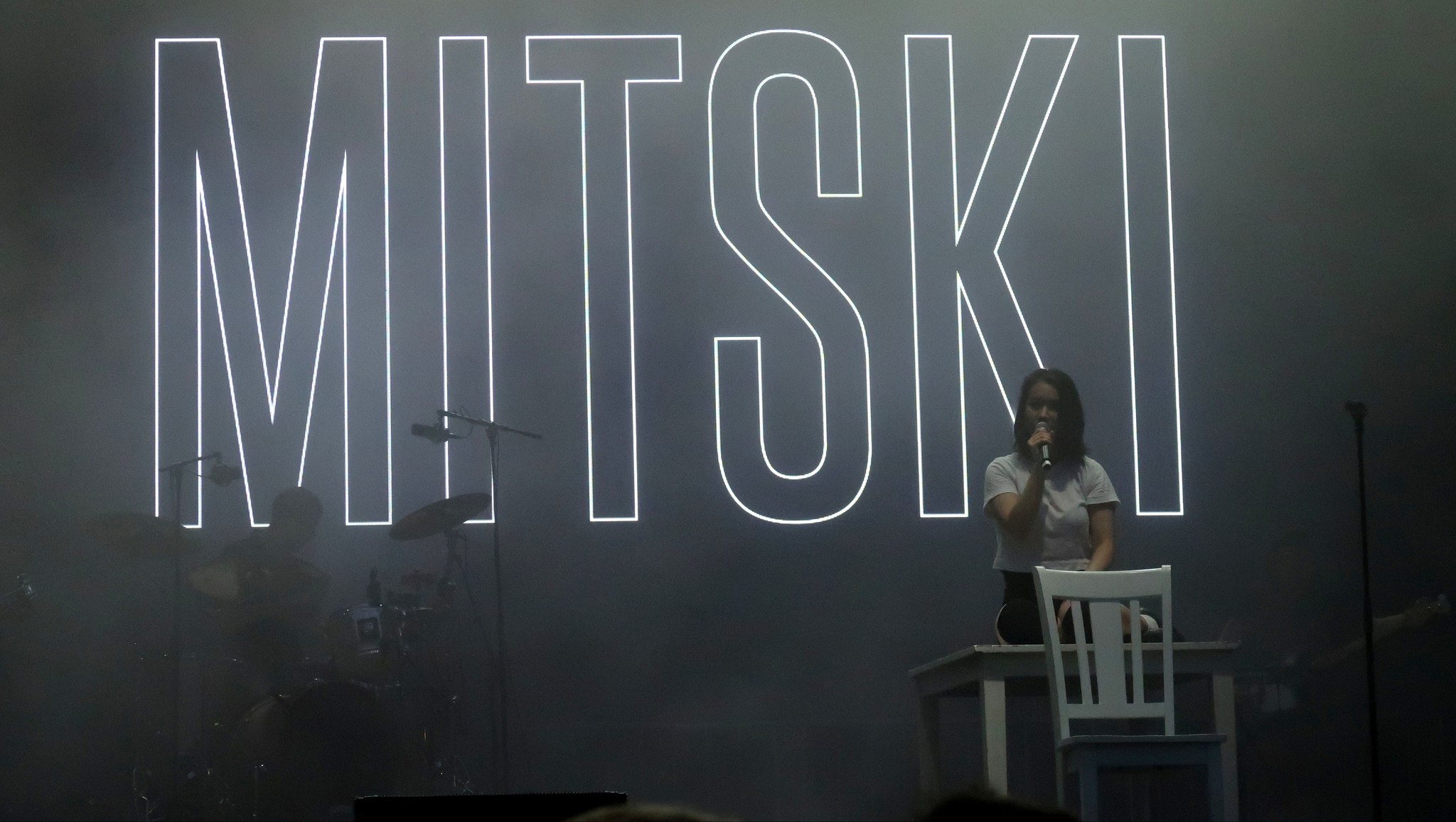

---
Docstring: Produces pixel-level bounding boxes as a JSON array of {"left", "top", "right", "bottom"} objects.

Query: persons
[
  {"left": 217, "top": 488, "right": 386, "bottom": 804},
  {"left": 979, "top": 368, "right": 1184, "bottom": 649}
]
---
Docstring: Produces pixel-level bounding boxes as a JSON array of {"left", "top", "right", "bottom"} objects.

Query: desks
[{"left": 907, "top": 642, "right": 1240, "bottom": 822}]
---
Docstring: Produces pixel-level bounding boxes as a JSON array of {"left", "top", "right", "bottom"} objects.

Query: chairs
[{"left": 1031, "top": 565, "right": 1228, "bottom": 822}]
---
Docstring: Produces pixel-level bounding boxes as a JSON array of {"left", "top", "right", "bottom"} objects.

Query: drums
[
  {"left": 227, "top": 679, "right": 394, "bottom": 803},
  {"left": 336, "top": 604, "right": 393, "bottom": 675}
]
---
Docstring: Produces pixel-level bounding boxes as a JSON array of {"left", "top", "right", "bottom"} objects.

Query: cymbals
[
  {"left": 387, "top": 492, "right": 492, "bottom": 540},
  {"left": 401, "top": 607, "right": 436, "bottom": 612},
  {"left": 189, "top": 557, "right": 327, "bottom": 604},
  {"left": 86, "top": 510, "right": 205, "bottom": 560}
]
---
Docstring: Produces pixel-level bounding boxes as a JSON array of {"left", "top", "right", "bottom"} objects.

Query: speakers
[{"left": 353, "top": 791, "right": 628, "bottom": 822}]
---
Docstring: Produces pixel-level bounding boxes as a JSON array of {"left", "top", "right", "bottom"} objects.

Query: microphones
[
  {"left": 208, "top": 468, "right": 243, "bottom": 482},
  {"left": 1034, "top": 421, "right": 1053, "bottom": 471},
  {"left": 366, "top": 568, "right": 382, "bottom": 600},
  {"left": 411, "top": 423, "right": 462, "bottom": 441}
]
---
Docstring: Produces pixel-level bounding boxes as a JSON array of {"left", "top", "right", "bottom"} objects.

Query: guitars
[{"left": 0, "top": 573, "right": 39, "bottom": 616}]
